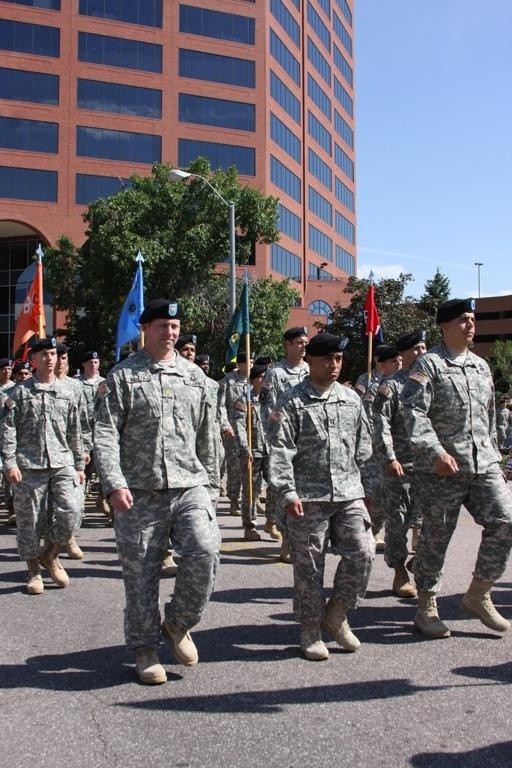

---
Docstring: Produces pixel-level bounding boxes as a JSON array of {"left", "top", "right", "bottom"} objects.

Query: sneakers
[
  {"left": 135, "top": 649, "right": 167, "bottom": 684},
  {"left": 161, "top": 619, "right": 200, "bottom": 666},
  {"left": 374, "top": 534, "right": 387, "bottom": 550},
  {"left": 160, "top": 555, "right": 179, "bottom": 574}
]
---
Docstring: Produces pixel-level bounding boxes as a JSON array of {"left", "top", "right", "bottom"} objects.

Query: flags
[
  {"left": 12, "top": 263, "right": 47, "bottom": 360},
  {"left": 114, "top": 265, "right": 145, "bottom": 362},
  {"left": 362, "top": 285, "right": 384, "bottom": 343},
  {"left": 223, "top": 282, "right": 250, "bottom": 365}
]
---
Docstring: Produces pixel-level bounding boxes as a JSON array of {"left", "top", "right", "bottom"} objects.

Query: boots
[
  {"left": 25, "top": 536, "right": 83, "bottom": 594},
  {"left": 411, "top": 527, "right": 422, "bottom": 552},
  {"left": 229, "top": 491, "right": 291, "bottom": 563},
  {"left": 415, "top": 591, "right": 450, "bottom": 638},
  {"left": 96, "top": 491, "right": 111, "bottom": 515},
  {"left": 321, "top": 599, "right": 361, "bottom": 652},
  {"left": 8, "top": 507, "right": 16, "bottom": 526},
  {"left": 461, "top": 578, "right": 511, "bottom": 631},
  {"left": 301, "top": 622, "right": 329, "bottom": 660},
  {"left": 393, "top": 564, "right": 417, "bottom": 597}
]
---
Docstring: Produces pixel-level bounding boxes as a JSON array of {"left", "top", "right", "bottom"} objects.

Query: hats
[
  {"left": 196, "top": 355, "right": 209, "bottom": 364},
  {"left": 284, "top": 326, "right": 308, "bottom": 339},
  {"left": 437, "top": 297, "right": 476, "bottom": 323},
  {"left": 139, "top": 299, "right": 181, "bottom": 324},
  {"left": 306, "top": 333, "right": 349, "bottom": 356},
  {"left": 374, "top": 330, "right": 428, "bottom": 360},
  {"left": 175, "top": 334, "right": 197, "bottom": 347},
  {"left": 224, "top": 352, "right": 270, "bottom": 378},
  {"left": 0, "top": 337, "right": 100, "bottom": 372}
]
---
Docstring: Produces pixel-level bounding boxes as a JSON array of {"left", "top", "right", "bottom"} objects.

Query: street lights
[
  {"left": 169, "top": 168, "right": 236, "bottom": 319},
  {"left": 473, "top": 260, "right": 487, "bottom": 301}
]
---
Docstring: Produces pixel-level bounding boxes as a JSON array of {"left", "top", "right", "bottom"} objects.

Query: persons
[
  {"left": 94, "top": 299, "right": 221, "bottom": 685},
  {"left": 337, "top": 329, "right": 427, "bottom": 599},
  {"left": 176, "top": 327, "right": 310, "bottom": 563},
  {"left": 265, "top": 333, "right": 373, "bottom": 661},
  {"left": 1, "top": 334, "right": 107, "bottom": 595},
  {"left": 496, "top": 400, "right": 510, "bottom": 451},
  {"left": 398, "top": 297, "right": 512, "bottom": 638}
]
